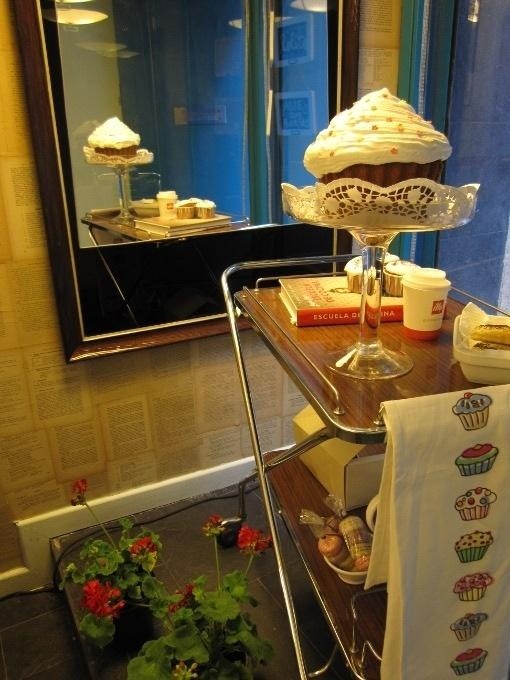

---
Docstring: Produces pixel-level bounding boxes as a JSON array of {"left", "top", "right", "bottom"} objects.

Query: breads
[
  {"left": 471, "top": 341, "right": 510, "bottom": 350},
  {"left": 469, "top": 324, "right": 510, "bottom": 344}
]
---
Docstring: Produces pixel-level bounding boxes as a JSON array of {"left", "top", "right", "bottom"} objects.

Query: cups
[
  {"left": 399, "top": 268, "right": 451, "bottom": 341},
  {"left": 156, "top": 191, "right": 178, "bottom": 219}
]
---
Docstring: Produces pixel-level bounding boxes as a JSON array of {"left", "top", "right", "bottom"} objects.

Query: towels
[{"left": 363, "top": 383, "right": 509, "bottom": 680}]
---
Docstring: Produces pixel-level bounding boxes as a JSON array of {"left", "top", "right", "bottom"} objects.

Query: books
[
  {"left": 277, "top": 275, "right": 449, "bottom": 329},
  {"left": 133, "top": 212, "right": 233, "bottom": 237}
]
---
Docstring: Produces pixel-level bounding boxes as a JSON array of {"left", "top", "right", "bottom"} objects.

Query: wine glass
[
  {"left": 277, "top": 189, "right": 480, "bottom": 383},
  {"left": 83, "top": 151, "right": 156, "bottom": 226}
]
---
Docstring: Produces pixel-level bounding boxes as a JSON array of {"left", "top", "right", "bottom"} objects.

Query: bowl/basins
[{"left": 320, "top": 530, "right": 376, "bottom": 586}]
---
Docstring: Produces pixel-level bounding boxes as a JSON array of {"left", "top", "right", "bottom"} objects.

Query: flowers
[
  {"left": 57, "top": 478, "right": 163, "bottom": 651},
  {"left": 127, "top": 517, "right": 272, "bottom": 680}
]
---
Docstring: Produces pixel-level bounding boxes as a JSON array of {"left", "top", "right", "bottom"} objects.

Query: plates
[
  {"left": 450, "top": 313, "right": 510, "bottom": 386},
  {"left": 128, "top": 199, "right": 160, "bottom": 218}
]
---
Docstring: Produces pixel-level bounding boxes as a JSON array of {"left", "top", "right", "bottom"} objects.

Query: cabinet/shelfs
[
  {"left": 80, "top": 208, "right": 231, "bottom": 327},
  {"left": 220, "top": 253, "right": 510, "bottom": 680}
]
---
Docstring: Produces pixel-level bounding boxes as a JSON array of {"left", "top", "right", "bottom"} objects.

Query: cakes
[
  {"left": 176, "top": 197, "right": 216, "bottom": 219},
  {"left": 302, "top": 86, "right": 452, "bottom": 184},
  {"left": 344, "top": 252, "right": 422, "bottom": 297},
  {"left": 318, "top": 516, "right": 375, "bottom": 571},
  {"left": 448, "top": 392, "right": 500, "bottom": 675},
  {"left": 87, "top": 117, "right": 140, "bottom": 158}
]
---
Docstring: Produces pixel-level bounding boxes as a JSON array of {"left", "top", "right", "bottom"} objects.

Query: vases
[
  {"left": 228, "top": 651, "right": 249, "bottom": 667},
  {"left": 114, "top": 571, "right": 157, "bottom": 642}
]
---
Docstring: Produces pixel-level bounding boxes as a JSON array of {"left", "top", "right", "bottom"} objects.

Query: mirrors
[{"left": 13, "top": 0, "right": 361, "bottom": 365}]
